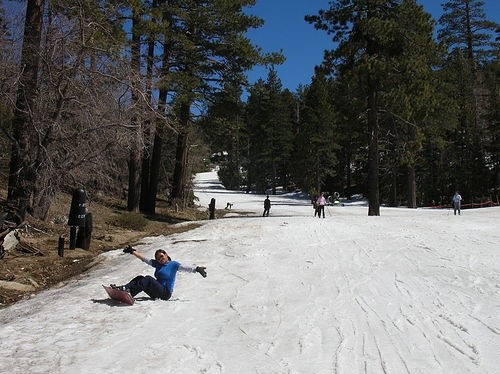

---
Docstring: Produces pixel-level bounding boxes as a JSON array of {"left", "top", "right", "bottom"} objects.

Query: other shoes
[{"left": 110, "top": 283, "right": 132, "bottom": 298}]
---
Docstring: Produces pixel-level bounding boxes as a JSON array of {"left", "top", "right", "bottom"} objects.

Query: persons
[
  {"left": 225, "top": 202, "right": 233, "bottom": 210},
  {"left": 316, "top": 193, "right": 326, "bottom": 218},
  {"left": 453, "top": 191, "right": 462, "bottom": 215},
  {"left": 313, "top": 196, "right": 319, "bottom": 217},
  {"left": 110, "top": 245, "right": 208, "bottom": 301},
  {"left": 263, "top": 195, "right": 271, "bottom": 217}
]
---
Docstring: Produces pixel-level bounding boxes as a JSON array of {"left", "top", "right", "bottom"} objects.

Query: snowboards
[{"left": 101, "top": 284, "right": 135, "bottom": 306}]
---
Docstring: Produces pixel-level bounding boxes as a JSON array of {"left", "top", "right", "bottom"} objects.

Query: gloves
[
  {"left": 122, "top": 244, "right": 137, "bottom": 254},
  {"left": 195, "top": 266, "right": 208, "bottom": 278}
]
